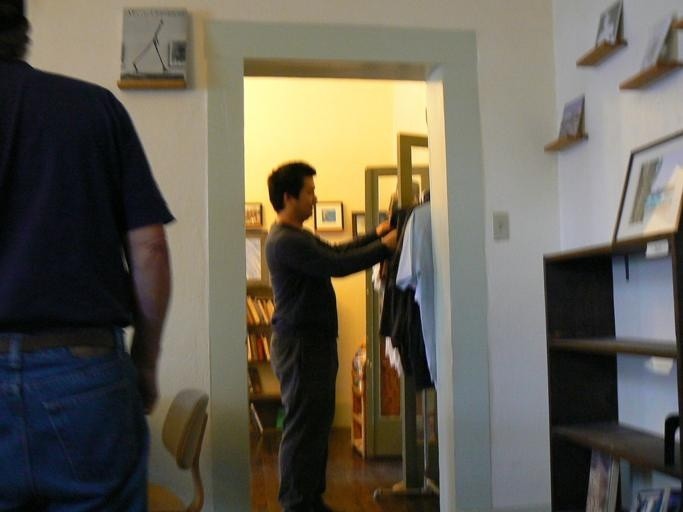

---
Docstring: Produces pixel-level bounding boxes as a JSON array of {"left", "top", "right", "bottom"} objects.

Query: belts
[{"left": 0, "top": 331, "right": 88, "bottom": 356}]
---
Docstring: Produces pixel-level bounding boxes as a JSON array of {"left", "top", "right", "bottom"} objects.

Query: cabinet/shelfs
[
  {"left": 544, "top": 233, "right": 683, "bottom": 512},
  {"left": 246, "top": 284, "right": 285, "bottom": 436},
  {"left": 349, "top": 346, "right": 424, "bottom": 462},
  {"left": 543, "top": 19, "right": 683, "bottom": 153}
]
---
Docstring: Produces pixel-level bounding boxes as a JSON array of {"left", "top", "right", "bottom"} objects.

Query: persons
[
  {"left": 0, "top": 0, "right": 177, "bottom": 511},
  {"left": 262, "top": 159, "right": 403, "bottom": 511}
]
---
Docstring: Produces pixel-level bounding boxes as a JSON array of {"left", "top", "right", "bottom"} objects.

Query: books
[
  {"left": 348, "top": 345, "right": 366, "bottom": 393},
  {"left": 246, "top": 292, "right": 276, "bottom": 436},
  {"left": 584, "top": 446, "right": 682, "bottom": 510}
]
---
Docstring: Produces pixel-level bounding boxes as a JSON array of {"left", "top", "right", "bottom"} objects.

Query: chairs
[{"left": 147, "top": 387, "right": 209, "bottom": 512}]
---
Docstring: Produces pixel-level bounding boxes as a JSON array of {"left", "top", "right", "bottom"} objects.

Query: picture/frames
[
  {"left": 595, "top": 1, "right": 623, "bottom": 44},
  {"left": 352, "top": 211, "right": 388, "bottom": 243},
  {"left": 314, "top": 200, "right": 344, "bottom": 232},
  {"left": 244, "top": 229, "right": 268, "bottom": 284},
  {"left": 611, "top": 127, "right": 683, "bottom": 248}
]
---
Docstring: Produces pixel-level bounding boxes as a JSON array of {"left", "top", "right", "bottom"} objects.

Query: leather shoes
[{"left": 283, "top": 495, "right": 336, "bottom": 512}]
